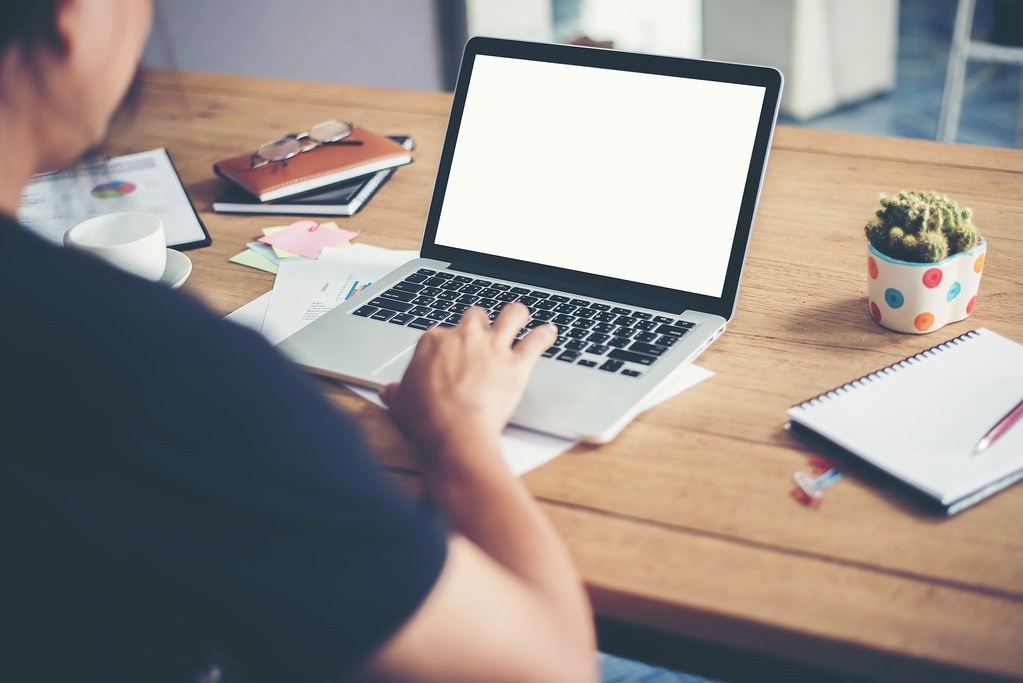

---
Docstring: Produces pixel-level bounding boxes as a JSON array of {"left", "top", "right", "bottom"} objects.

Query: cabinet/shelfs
[{"left": 704, "top": 1, "right": 903, "bottom": 123}]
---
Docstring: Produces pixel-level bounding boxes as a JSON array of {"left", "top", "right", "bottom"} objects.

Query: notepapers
[{"left": 229, "top": 220, "right": 360, "bottom": 275}]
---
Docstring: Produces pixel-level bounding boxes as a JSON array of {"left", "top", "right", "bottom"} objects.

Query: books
[
  {"left": 784, "top": 327, "right": 1023, "bottom": 517},
  {"left": 211, "top": 114, "right": 415, "bottom": 218},
  {"left": 18, "top": 146, "right": 212, "bottom": 252}
]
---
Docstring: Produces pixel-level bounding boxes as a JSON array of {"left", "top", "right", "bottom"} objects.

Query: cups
[{"left": 63, "top": 210, "right": 168, "bottom": 283}]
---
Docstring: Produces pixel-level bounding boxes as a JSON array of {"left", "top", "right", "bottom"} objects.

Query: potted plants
[{"left": 864, "top": 191, "right": 986, "bottom": 333}]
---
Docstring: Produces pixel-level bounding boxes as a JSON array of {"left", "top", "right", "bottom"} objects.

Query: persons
[{"left": 0, "top": 0, "right": 599, "bottom": 682}]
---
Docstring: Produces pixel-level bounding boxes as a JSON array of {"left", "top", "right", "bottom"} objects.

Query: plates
[{"left": 157, "top": 247, "right": 194, "bottom": 292}]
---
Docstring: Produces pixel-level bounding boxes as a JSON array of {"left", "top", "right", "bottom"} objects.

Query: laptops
[{"left": 272, "top": 35, "right": 784, "bottom": 444}]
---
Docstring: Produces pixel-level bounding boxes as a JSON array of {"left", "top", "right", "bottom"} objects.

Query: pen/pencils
[{"left": 972, "top": 400, "right": 1022, "bottom": 455}]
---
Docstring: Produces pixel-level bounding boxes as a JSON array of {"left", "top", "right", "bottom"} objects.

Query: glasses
[{"left": 249, "top": 119, "right": 363, "bottom": 170}]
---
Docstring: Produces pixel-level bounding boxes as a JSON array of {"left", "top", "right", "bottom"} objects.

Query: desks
[{"left": 17, "top": 65, "right": 1023, "bottom": 683}]
